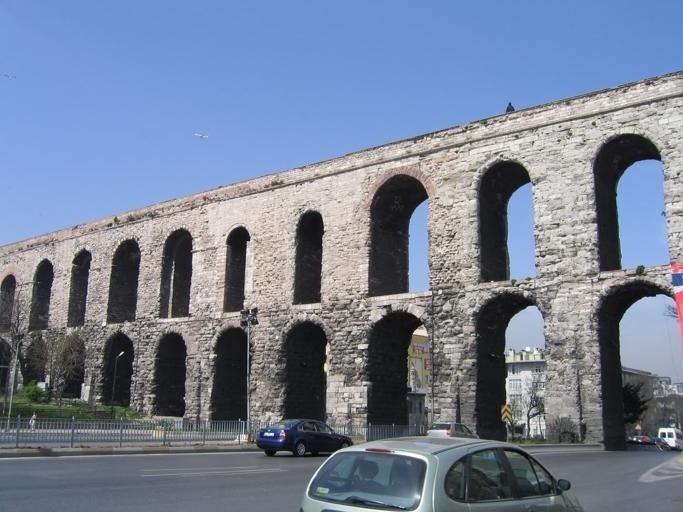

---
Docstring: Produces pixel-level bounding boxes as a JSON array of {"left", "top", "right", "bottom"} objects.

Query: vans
[{"left": 658, "top": 428, "right": 683, "bottom": 450}]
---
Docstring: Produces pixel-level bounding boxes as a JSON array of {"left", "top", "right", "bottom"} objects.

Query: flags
[{"left": 671, "top": 265, "right": 683, "bottom": 337}]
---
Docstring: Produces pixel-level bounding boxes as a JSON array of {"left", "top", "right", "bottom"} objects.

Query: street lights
[
  {"left": 110, "top": 351, "right": 126, "bottom": 405},
  {"left": 238, "top": 307, "right": 258, "bottom": 420}
]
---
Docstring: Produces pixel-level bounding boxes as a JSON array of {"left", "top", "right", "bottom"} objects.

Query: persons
[{"left": 29, "top": 412, "right": 37, "bottom": 430}]
